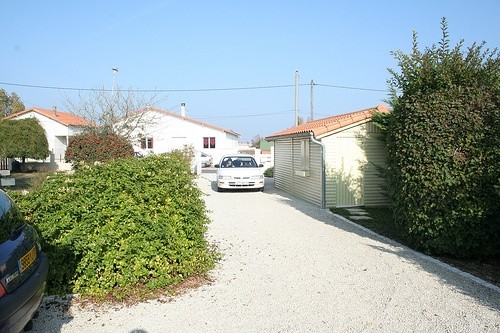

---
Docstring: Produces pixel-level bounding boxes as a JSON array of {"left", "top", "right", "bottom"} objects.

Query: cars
[
  {"left": 216, "top": 154, "right": 265, "bottom": 192},
  {"left": 0, "top": 187, "right": 49, "bottom": 333},
  {"left": 201, "top": 152, "right": 213, "bottom": 168}
]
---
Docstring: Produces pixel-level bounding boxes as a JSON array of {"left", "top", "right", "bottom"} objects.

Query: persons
[{"left": 226, "top": 159, "right": 232, "bottom": 167}]
